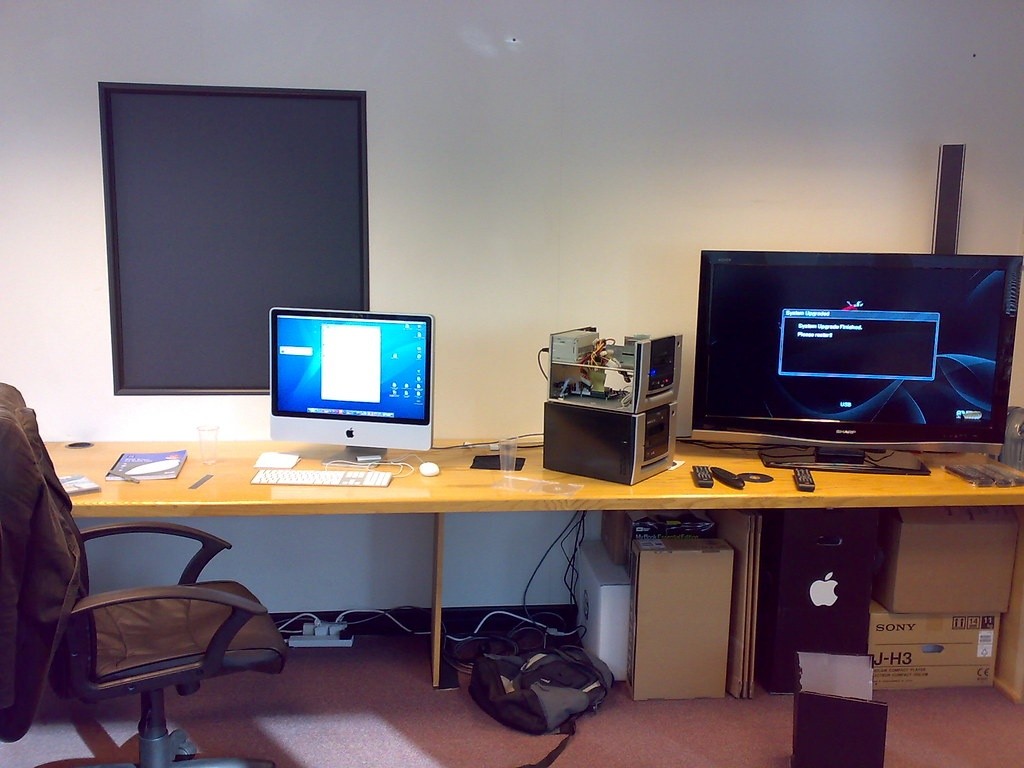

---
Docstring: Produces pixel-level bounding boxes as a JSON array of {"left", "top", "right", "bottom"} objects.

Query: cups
[{"left": 498, "top": 439, "right": 517, "bottom": 472}]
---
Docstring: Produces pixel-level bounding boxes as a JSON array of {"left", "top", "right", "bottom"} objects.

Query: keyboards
[{"left": 250, "top": 469, "right": 391, "bottom": 487}]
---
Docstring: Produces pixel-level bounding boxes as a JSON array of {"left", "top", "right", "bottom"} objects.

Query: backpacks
[{"left": 468, "top": 644, "right": 615, "bottom": 768}]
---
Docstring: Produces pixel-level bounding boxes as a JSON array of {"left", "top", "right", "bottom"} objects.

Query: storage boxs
[
  {"left": 626, "top": 536, "right": 732, "bottom": 702},
  {"left": 875, "top": 507, "right": 1019, "bottom": 614},
  {"left": 573, "top": 536, "right": 628, "bottom": 683},
  {"left": 596, "top": 511, "right": 626, "bottom": 563},
  {"left": 626, "top": 511, "right": 717, "bottom": 538},
  {"left": 867, "top": 600, "right": 1008, "bottom": 691}
]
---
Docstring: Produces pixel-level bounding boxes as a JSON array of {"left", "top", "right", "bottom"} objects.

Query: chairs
[{"left": 0, "top": 382, "right": 298, "bottom": 768}]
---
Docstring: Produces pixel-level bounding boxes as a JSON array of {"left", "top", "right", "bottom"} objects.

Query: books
[
  {"left": 58, "top": 475, "right": 101, "bottom": 497},
  {"left": 106, "top": 450, "right": 188, "bottom": 481}
]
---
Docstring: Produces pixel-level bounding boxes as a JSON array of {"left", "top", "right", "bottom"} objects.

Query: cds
[{"left": 736, "top": 472, "right": 774, "bottom": 483}]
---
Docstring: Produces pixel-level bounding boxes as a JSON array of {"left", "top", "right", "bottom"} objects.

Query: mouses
[{"left": 418, "top": 462, "right": 439, "bottom": 476}]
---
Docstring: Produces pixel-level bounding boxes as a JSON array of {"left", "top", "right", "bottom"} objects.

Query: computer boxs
[{"left": 543, "top": 332, "right": 686, "bottom": 486}]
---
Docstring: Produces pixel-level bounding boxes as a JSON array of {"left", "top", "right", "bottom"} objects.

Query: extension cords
[{"left": 288, "top": 633, "right": 355, "bottom": 647}]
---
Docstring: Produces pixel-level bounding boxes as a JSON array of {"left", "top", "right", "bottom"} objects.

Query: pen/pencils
[{"left": 109, "top": 469, "right": 141, "bottom": 484}]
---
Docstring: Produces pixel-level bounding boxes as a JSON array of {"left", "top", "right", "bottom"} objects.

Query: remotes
[
  {"left": 792, "top": 467, "right": 815, "bottom": 492},
  {"left": 946, "top": 464, "right": 1024, "bottom": 488},
  {"left": 691, "top": 465, "right": 713, "bottom": 488},
  {"left": 710, "top": 466, "right": 746, "bottom": 488}
]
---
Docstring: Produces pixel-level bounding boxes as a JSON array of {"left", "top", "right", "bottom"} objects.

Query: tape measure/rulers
[{"left": 187, "top": 474, "right": 214, "bottom": 489}]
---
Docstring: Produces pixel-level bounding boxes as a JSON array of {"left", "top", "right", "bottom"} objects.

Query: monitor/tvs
[
  {"left": 268, "top": 307, "right": 436, "bottom": 467},
  {"left": 687, "top": 249, "right": 1023, "bottom": 474}
]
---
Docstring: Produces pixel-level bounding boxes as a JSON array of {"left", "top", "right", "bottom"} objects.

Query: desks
[{"left": 44, "top": 429, "right": 1024, "bottom": 686}]
